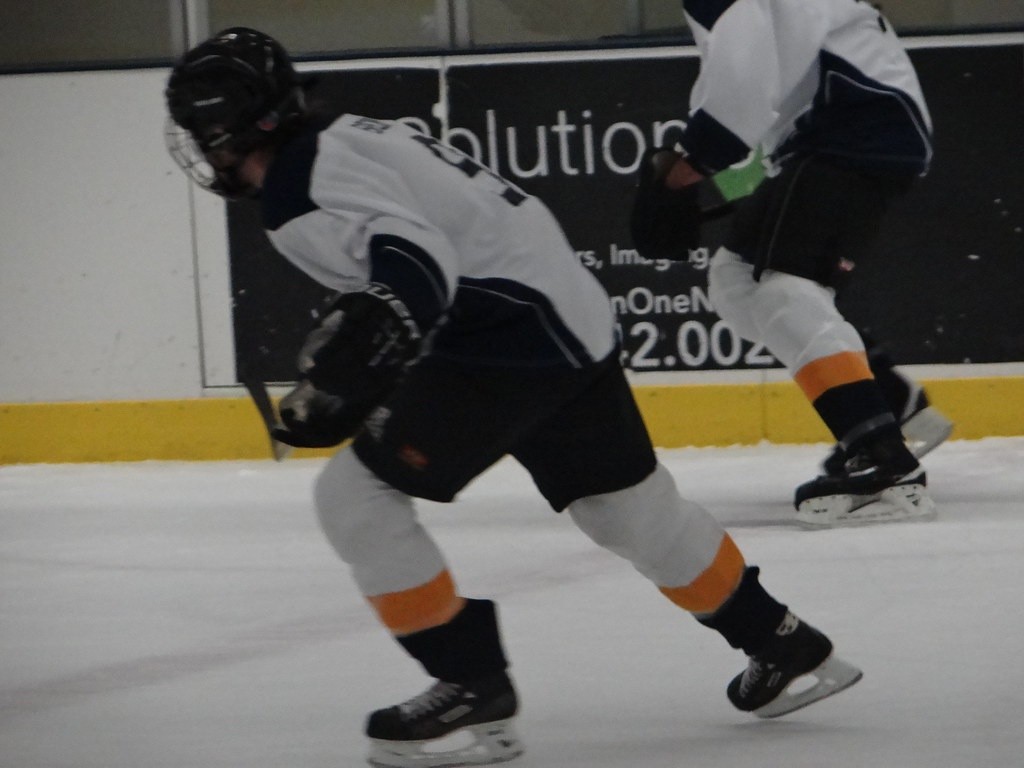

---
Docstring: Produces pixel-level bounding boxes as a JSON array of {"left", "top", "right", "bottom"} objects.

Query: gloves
[
  {"left": 631, "top": 148, "right": 702, "bottom": 263},
  {"left": 296, "top": 277, "right": 436, "bottom": 403},
  {"left": 269, "top": 382, "right": 361, "bottom": 449}
]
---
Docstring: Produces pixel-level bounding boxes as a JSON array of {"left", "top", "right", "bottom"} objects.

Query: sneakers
[
  {"left": 821, "top": 369, "right": 953, "bottom": 474},
  {"left": 725, "top": 608, "right": 863, "bottom": 718},
  {"left": 365, "top": 668, "right": 521, "bottom": 767},
  {"left": 795, "top": 411, "right": 935, "bottom": 524}
]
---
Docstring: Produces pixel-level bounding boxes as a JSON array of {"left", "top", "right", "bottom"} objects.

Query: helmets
[{"left": 163, "top": 22, "right": 300, "bottom": 204}]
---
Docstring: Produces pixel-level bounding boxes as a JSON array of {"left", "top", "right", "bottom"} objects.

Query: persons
[
  {"left": 165, "top": 26, "right": 861, "bottom": 768},
  {"left": 631, "top": 0, "right": 953, "bottom": 527}
]
